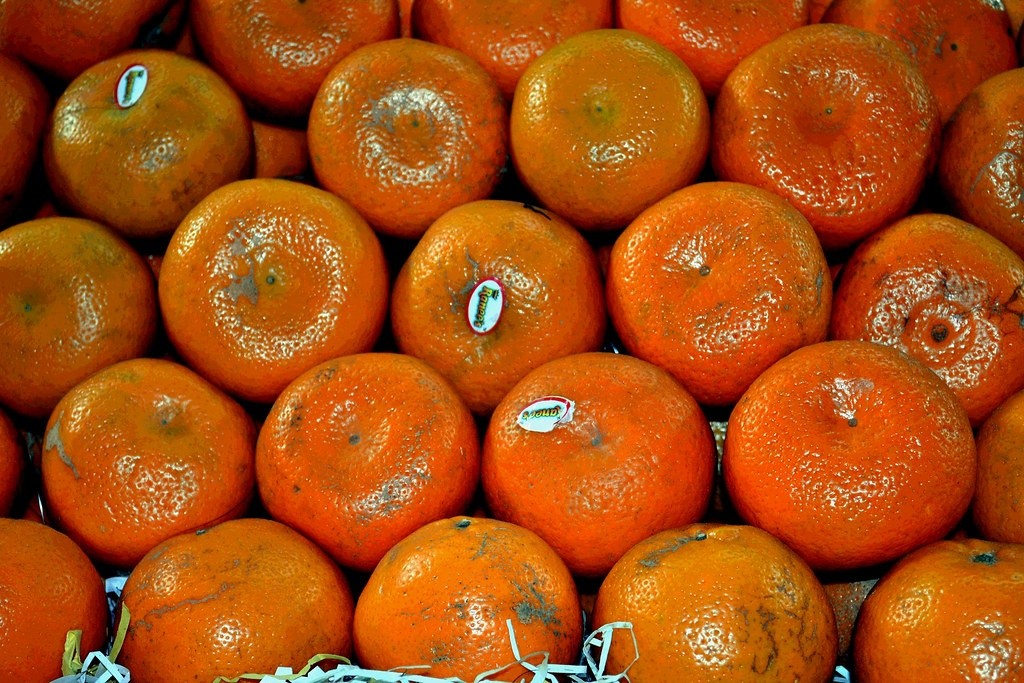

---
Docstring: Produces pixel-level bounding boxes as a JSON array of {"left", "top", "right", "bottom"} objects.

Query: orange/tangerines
[{"left": 0, "top": 0, "right": 1024, "bottom": 683}]
